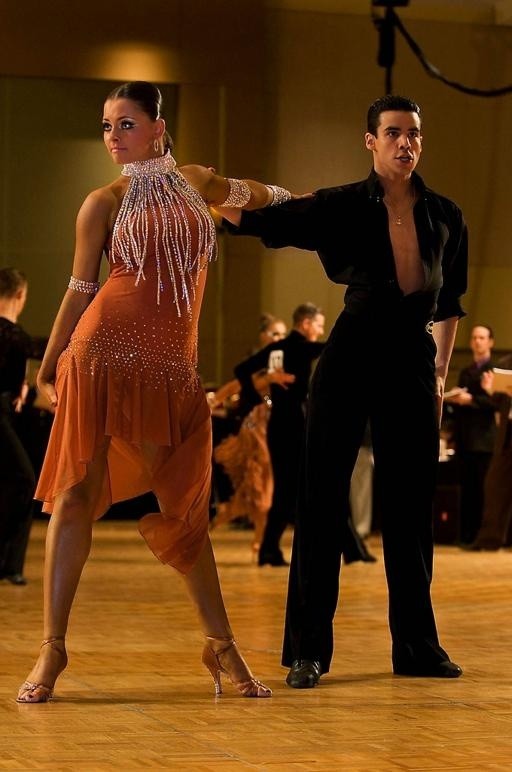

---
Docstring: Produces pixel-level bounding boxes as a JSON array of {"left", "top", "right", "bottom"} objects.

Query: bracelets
[
  {"left": 266, "top": 181, "right": 291, "bottom": 208},
  {"left": 66, "top": 276, "right": 101, "bottom": 295}
]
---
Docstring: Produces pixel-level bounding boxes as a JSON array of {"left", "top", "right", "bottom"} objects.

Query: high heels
[
  {"left": 16, "top": 635, "right": 68, "bottom": 704},
  {"left": 202, "top": 635, "right": 272, "bottom": 698}
]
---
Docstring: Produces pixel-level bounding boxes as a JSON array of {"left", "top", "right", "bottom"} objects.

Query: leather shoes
[
  {"left": 4, "top": 573, "right": 26, "bottom": 584},
  {"left": 418, "top": 660, "right": 463, "bottom": 678},
  {"left": 286, "top": 658, "right": 323, "bottom": 689}
]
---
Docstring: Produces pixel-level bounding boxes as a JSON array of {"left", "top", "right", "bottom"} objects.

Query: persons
[
  {"left": 204, "top": 303, "right": 511, "bottom": 565},
  {"left": 12, "top": 80, "right": 316, "bottom": 705},
  {"left": 0, "top": 268, "right": 37, "bottom": 587},
  {"left": 207, "top": 93, "right": 469, "bottom": 690},
  {"left": 21, "top": 363, "right": 58, "bottom": 520}
]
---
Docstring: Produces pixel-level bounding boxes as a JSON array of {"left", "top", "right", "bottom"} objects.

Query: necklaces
[{"left": 383, "top": 199, "right": 412, "bottom": 226}]
